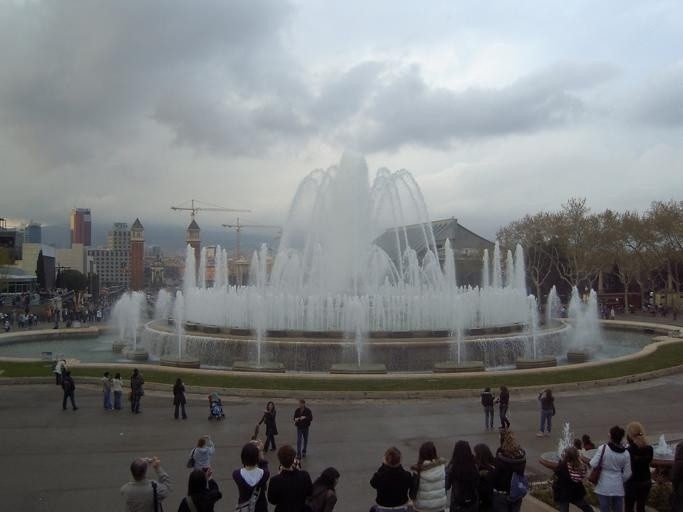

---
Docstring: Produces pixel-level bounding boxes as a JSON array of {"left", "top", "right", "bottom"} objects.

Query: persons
[
  {"left": 481, "top": 386, "right": 495, "bottom": 429},
  {"left": 187, "top": 436, "right": 216, "bottom": 476},
  {"left": 112, "top": 373, "right": 123, "bottom": 408},
  {"left": 61, "top": 371, "right": 78, "bottom": 410},
  {"left": 497, "top": 385, "right": 511, "bottom": 429},
  {"left": 129, "top": 369, "right": 143, "bottom": 414},
  {"left": 261, "top": 401, "right": 278, "bottom": 452},
  {"left": 369, "top": 431, "right": 528, "bottom": 512},
  {"left": 120, "top": 456, "right": 172, "bottom": 512},
  {"left": 102, "top": 372, "right": 112, "bottom": 409},
  {"left": 294, "top": 399, "right": 313, "bottom": 460},
  {"left": 303, "top": 466, "right": 341, "bottom": 511},
  {"left": 0, "top": 287, "right": 110, "bottom": 332},
  {"left": 535, "top": 389, "right": 555, "bottom": 435},
  {"left": 172, "top": 379, "right": 187, "bottom": 419},
  {"left": 231, "top": 441, "right": 270, "bottom": 511},
  {"left": 550, "top": 422, "right": 683, "bottom": 512},
  {"left": 176, "top": 468, "right": 223, "bottom": 512},
  {"left": 559, "top": 294, "right": 678, "bottom": 322},
  {"left": 267, "top": 443, "right": 314, "bottom": 511},
  {"left": 53, "top": 360, "right": 66, "bottom": 385}
]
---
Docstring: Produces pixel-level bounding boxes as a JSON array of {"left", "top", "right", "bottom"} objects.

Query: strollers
[{"left": 207, "top": 391, "right": 226, "bottom": 421}]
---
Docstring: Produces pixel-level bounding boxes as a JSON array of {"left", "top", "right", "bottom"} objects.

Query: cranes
[
  {"left": 169, "top": 198, "right": 254, "bottom": 221},
  {"left": 219, "top": 214, "right": 283, "bottom": 257}
]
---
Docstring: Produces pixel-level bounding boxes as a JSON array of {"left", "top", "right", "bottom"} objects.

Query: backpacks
[{"left": 507, "top": 471, "right": 529, "bottom": 503}]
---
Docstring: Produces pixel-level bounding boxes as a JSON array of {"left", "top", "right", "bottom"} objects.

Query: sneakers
[
  {"left": 536, "top": 432, "right": 550, "bottom": 438},
  {"left": 506, "top": 423, "right": 510, "bottom": 428},
  {"left": 498, "top": 426, "right": 505, "bottom": 429},
  {"left": 270, "top": 447, "right": 277, "bottom": 451}
]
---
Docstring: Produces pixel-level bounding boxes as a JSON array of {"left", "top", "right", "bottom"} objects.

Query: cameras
[
  {"left": 146, "top": 458, "right": 154, "bottom": 464},
  {"left": 250, "top": 439, "right": 260, "bottom": 446}
]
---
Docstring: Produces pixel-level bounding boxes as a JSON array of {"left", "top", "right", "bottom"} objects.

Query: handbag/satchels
[
  {"left": 234, "top": 496, "right": 259, "bottom": 512},
  {"left": 250, "top": 427, "right": 260, "bottom": 441},
  {"left": 552, "top": 406, "right": 555, "bottom": 416},
  {"left": 587, "top": 463, "right": 602, "bottom": 486},
  {"left": 186, "top": 457, "right": 195, "bottom": 468}
]
